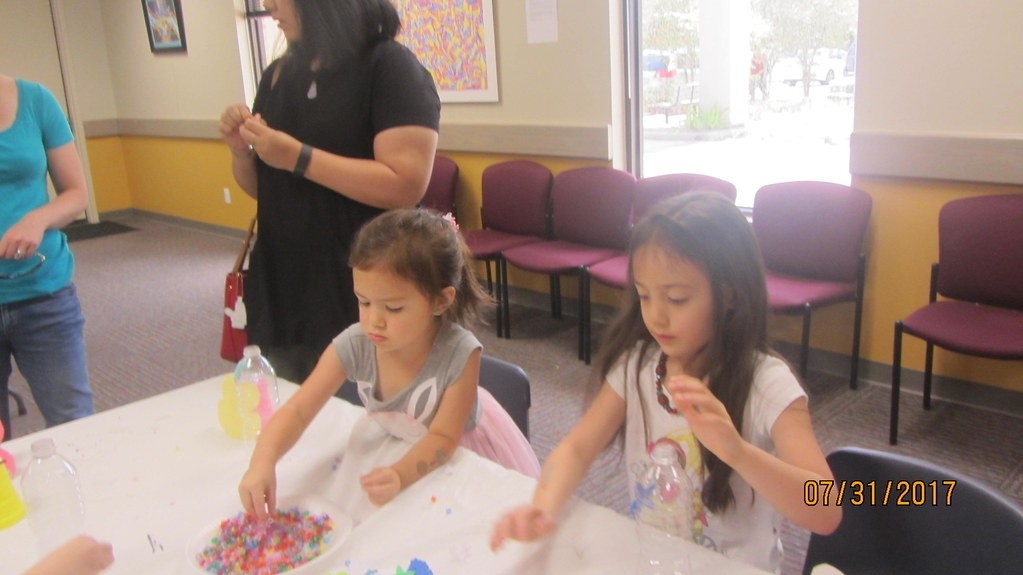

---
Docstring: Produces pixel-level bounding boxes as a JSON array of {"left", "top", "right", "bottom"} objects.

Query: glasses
[{"left": 0, "top": 253, "right": 46, "bottom": 279}]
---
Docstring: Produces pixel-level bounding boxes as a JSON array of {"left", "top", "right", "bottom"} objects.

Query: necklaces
[{"left": 655, "top": 354, "right": 681, "bottom": 415}]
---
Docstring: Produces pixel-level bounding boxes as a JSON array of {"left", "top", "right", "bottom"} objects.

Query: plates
[{"left": 184, "top": 499, "right": 353, "bottom": 575}]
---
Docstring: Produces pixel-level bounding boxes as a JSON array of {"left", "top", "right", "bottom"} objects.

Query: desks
[{"left": 0, "top": 372, "right": 771, "bottom": 575}]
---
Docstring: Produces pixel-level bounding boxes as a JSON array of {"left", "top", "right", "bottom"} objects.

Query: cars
[
  {"left": 640, "top": 47, "right": 699, "bottom": 110},
  {"left": 769, "top": 47, "right": 846, "bottom": 86}
]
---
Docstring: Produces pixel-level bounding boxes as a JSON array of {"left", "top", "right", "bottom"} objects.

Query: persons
[
  {"left": 490, "top": 189, "right": 841, "bottom": 575},
  {"left": 218, "top": 0, "right": 442, "bottom": 407},
  {"left": 0, "top": 74, "right": 93, "bottom": 442},
  {"left": 749, "top": 53, "right": 766, "bottom": 100},
  {"left": 237, "top": 209, "right": 541, "bottom": 520},
  {"left": 24, "top": 535, "right": 113, "bottom": 575}
]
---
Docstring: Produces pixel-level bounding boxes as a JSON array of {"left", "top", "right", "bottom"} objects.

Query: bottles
[
  {"left": 21, "top": 438, "right": 84, "bottom": 555},
  {"left": 233, "top": 345, "right": 281, "bottom": 458},
  {"left": 631, "top": 445, "right": 694, "bottom": 575}
]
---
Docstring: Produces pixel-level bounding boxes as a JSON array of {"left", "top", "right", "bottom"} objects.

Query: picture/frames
[
  {"left": 390, "top": 0, "right": 500, "bottom": 104},
  {"left": 141, "top": 0, "right": 187, "bottom": 53}
]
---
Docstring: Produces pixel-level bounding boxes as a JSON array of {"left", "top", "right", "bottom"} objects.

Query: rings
[{"left": 16, "top": 249, "right": 25, "bottom": 257}]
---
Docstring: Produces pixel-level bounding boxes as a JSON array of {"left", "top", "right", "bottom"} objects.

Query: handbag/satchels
[{"left": 220, "top": 216, "right": 256, "bottom": 363}]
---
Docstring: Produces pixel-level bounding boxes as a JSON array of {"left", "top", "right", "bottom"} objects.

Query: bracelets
[{"left": 292, "top": 144, "right": 313, "bottom": 179}]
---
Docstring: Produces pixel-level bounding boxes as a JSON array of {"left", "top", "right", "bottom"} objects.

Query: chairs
[
  {"left": 478, "top": 355, "right": 532, "bottom": 442},
  {"left": 417, "top": 156, "right": 460, "bottom": 224},
  {"left": 890, "top": 193, "right": 1023, "bottom": 445},
  {"left": 751, "top": 181, "right": 873, "bottom": 393},
  {"left": 464, "top": 161, "right": 559, "bottom": 337},
  {"left": 583, "top": 172, "right": 736, "bottom": 365},
  {"left": 802, "top": 447, "right": 1023, "bottom": 575},
  {"left": 502, "top": 166, "right": 638, "bottom": 360}
]
